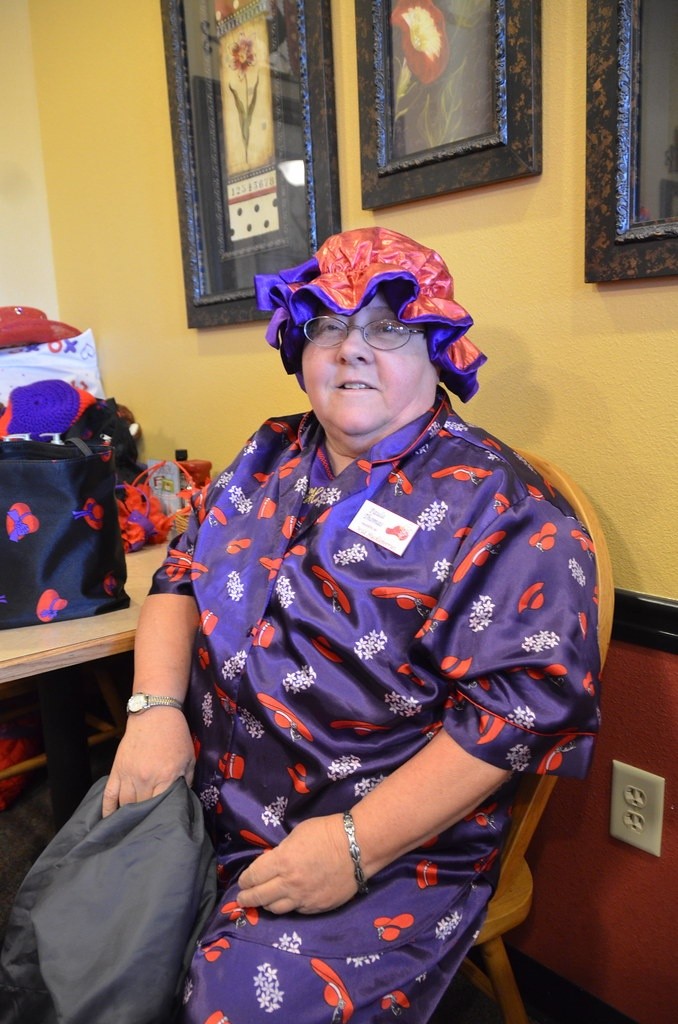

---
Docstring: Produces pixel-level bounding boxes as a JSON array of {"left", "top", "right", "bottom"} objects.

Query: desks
[{"left": 0, "top": 541, "right": 172, "bottom": 684}]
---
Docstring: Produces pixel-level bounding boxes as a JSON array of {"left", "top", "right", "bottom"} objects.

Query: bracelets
[{"left": 344, "top": 808, "right": 367, "bottom": 896}]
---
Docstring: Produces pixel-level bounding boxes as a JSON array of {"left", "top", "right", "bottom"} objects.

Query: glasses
[{"left": 301, "top": 314, "right": 423, "bottom": 352}]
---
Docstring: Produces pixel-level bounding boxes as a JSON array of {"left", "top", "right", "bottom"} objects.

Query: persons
[{"left": 101, "top": 228, "right": 599, "bottom": 1024}]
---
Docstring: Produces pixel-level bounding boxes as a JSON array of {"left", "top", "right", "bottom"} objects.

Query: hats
[
  {"left": 0, "top": 306, "right": 82, "bottom": 349},
  {"left": 253, "top": 227, "right": 488, "bottom": 403}
]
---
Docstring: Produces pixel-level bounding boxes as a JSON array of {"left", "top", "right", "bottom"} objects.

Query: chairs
[{"left": 459, "top": 449, "right": 616, "bottom": 1024}]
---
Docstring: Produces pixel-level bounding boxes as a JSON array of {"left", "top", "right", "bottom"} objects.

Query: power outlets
[{"left": 609, "top": 758, "right": 665, "bottom": 858}]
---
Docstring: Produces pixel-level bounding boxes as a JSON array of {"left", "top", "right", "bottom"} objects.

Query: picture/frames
[
  {"left": 160, "top": 0, "right": 343, "bottom": 331},
  {"left": 354, "top": 0, "right": 543, "bottom": 211},
  {"left": 582, "top": 0, "right": 678, "bottom": 285}
]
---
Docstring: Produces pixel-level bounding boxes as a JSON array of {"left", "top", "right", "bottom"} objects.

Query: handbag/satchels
[{"left": 1, "top": 440, "right": 132, "bottom": 629}]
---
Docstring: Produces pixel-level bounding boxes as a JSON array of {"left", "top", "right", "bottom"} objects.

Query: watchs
[{"left": 125, "top": 694, "right": 182, "bottom": 711}]
